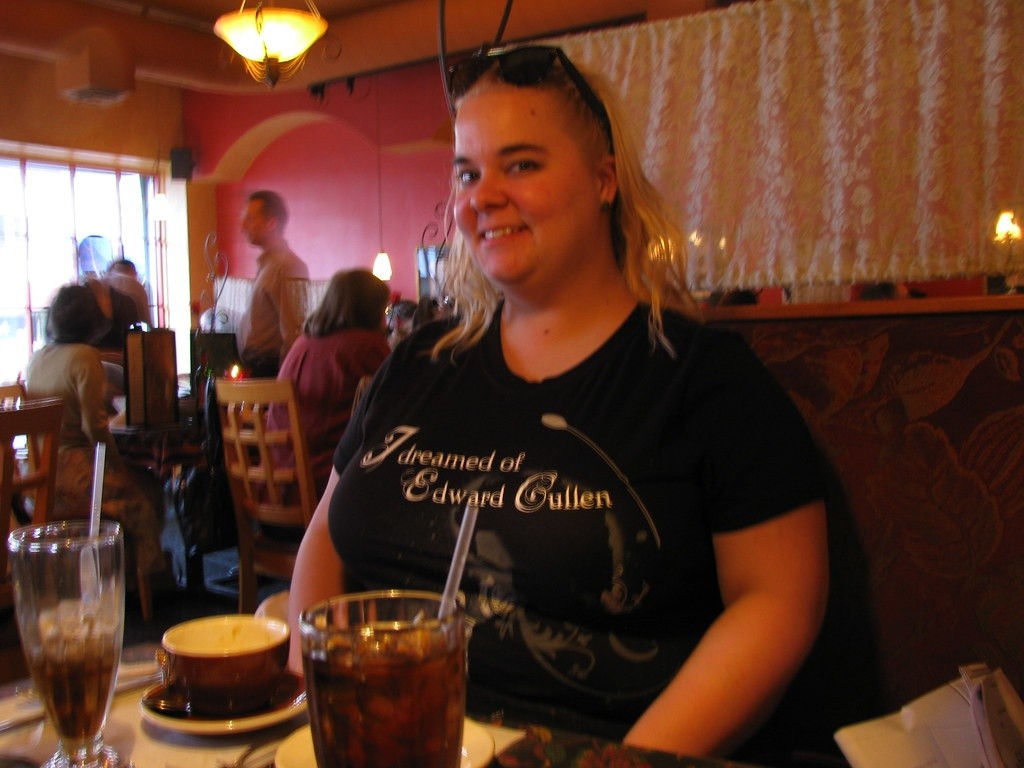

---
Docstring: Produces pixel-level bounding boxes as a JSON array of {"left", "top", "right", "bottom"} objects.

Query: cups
[
  {"left": 298, "top": 590, "right": 464, "bottom": 768},
  {"left": 6, "top": 520, "right": 124, "bottom": 768}
]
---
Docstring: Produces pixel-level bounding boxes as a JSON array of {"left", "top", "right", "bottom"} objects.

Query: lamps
[{"left": 213, "top": 0, "right": 331, "bottom": 87}]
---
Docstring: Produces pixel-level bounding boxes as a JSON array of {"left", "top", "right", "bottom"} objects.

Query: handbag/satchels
[{"left": 172, "top": 379, "right": 239, "bottom": 561}]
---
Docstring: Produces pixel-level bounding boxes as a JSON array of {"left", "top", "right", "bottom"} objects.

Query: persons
[
  {"left": 262, "top": 266, "right": 392, "bottom": 548},
  {"left": 24, "top": 259, "right": 194, "bottom": 626},
  {"left": 388, "top": 294, "right": 443, "bottom": 348},
  {"left": 228, "top": 190, "right": 310, "bottom": 576},
  {"left": 287, "top": 43, "right": 830, "bottom": 768}
]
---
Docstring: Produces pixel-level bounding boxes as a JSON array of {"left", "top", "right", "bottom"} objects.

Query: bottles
[{"left": 195, "top": 347, "right": 214, "bottom": 420}]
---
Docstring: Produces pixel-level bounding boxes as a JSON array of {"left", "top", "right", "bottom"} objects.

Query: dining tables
[
  {"left": 103, "top": 369, "right": 241, "bottom": 577},
  {"left": 1, "top": 618, "right": 751, "bottom": 768}
]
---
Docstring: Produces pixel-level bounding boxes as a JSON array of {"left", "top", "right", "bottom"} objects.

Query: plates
[
  {"left": 274, "top": 714, "right": 494, "bottom": 768},
  {"left": 139, "top": 671, "right": 309, "bottom": 734}
]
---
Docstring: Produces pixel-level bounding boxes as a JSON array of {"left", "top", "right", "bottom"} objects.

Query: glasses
[{"left": 445, "top": 44, "right": 614, "bottom": 153}]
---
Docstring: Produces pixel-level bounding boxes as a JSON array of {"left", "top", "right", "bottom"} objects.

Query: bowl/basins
[{"left": 162, "top": 614, "right": 291, "bottom": 699}]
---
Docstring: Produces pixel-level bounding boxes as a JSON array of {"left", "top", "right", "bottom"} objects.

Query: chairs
[{"left": 1, "top": 365, "right": 329, "bottom": 626}]
